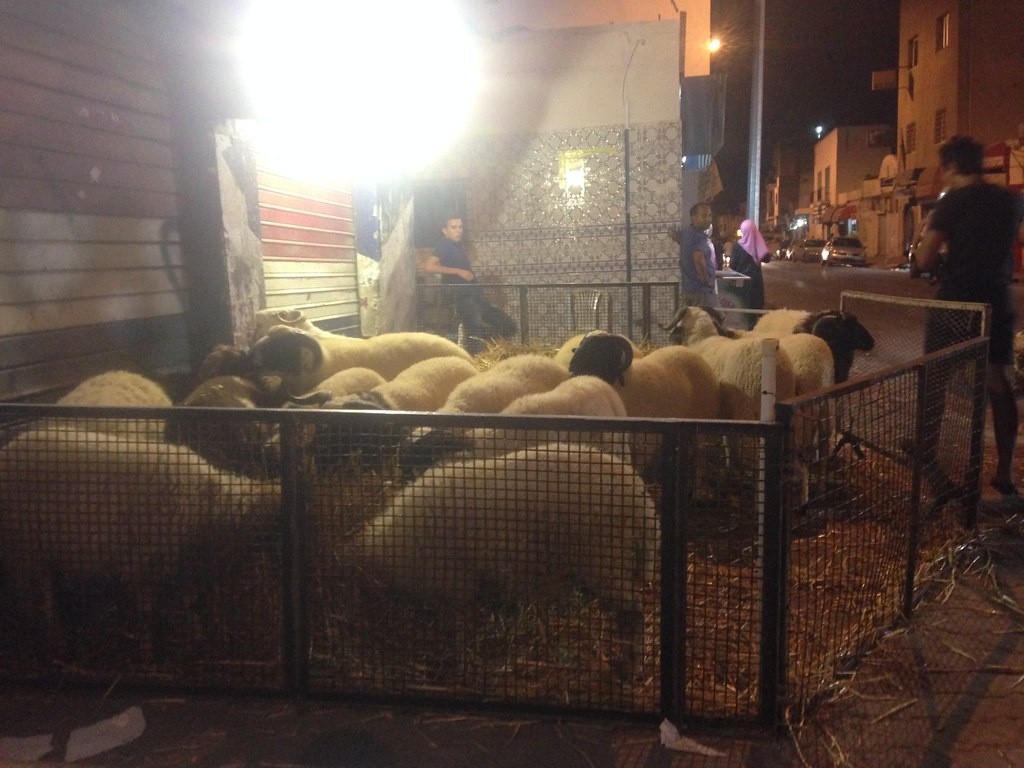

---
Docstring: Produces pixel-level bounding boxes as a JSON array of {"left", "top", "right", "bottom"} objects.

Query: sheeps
[
  {"left": 1, "top": 427, "right": 285, "bottom": 664},
  {"left": 658, "top": 306, "right": 837, "bottom": 503},
  {"left": 243, "top": 322, "right": 478, "bottom": 411},
  {"left": 750, "top": 307, "right": 880, "bottom": 384},
  {"left": 166, "top": 339, "right": 282, "bottom": 485},
  {"left": 270, "top": 353, "right": 632, "bottom": 475},
  {"left": 26, "top": 369, "right": 172, "bottom": 443},
  {"left": 557, "top": 333, "right": 726, "bottom": 502},
  {"left": 323, "top": 443, "right": 659, "bottom": 700}
]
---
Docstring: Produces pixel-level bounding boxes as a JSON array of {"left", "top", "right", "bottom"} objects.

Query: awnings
[
  {"left": 820, "top": 205, "right": 856, "bottom": 223},
  {"left": 916, "top": 164, "right": 944, "bottom": 199}
]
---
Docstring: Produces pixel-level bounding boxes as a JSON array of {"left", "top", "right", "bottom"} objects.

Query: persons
[
  {"left": 669, "top": 204, "right": 717, "bottom": 309},
  {"left": 423, "top": 216, "right": 486, "bottom": 357},
  {"left": 903, "top": 133, "right": 1024, "bottom": 498},
  {"left": 729, "top": 220, "right": 771, "bottom": 325}
]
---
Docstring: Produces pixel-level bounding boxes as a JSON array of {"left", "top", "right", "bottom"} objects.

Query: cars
[
  {"left": 820, "top": 236, "right": 867, "bottom": 266},
  {"left": 776, "top": 238, "right": 827, "bottom": 262}
]
